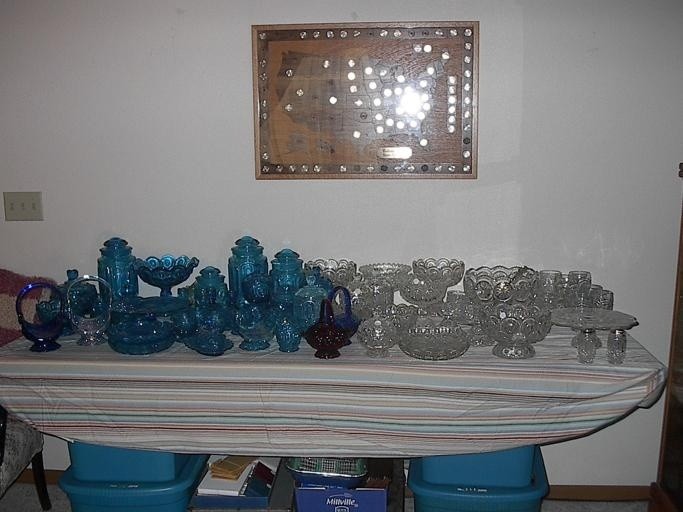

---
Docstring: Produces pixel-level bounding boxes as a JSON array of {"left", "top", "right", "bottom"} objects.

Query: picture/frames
[{"left": 250, "top": 20, "right": 480, "bottom": 181}]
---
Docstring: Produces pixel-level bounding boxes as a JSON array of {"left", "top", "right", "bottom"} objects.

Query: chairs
[{"left": 0, "top": 267, "right": 54, "bottom": 512}]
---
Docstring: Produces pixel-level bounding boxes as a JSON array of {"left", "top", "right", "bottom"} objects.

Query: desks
[{"left": 1, "top": 319, "right": 669, "bottom": 510}]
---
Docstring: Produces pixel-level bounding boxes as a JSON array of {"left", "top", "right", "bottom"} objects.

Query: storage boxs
[
  {"left": 407, "top": 446, "right": 550, "bottom": 512},
  {"left": 292, "top": 480, "right": 390, "bottom": 512},
  {"left": 421, "top": 445, "right": 536, "bottom": 490},
  {"left": 58, "top": 454, "right": 211, "bottom": 511},
  {"left": 67, "top": 439, "right": 193, "bottom": 483}
]
